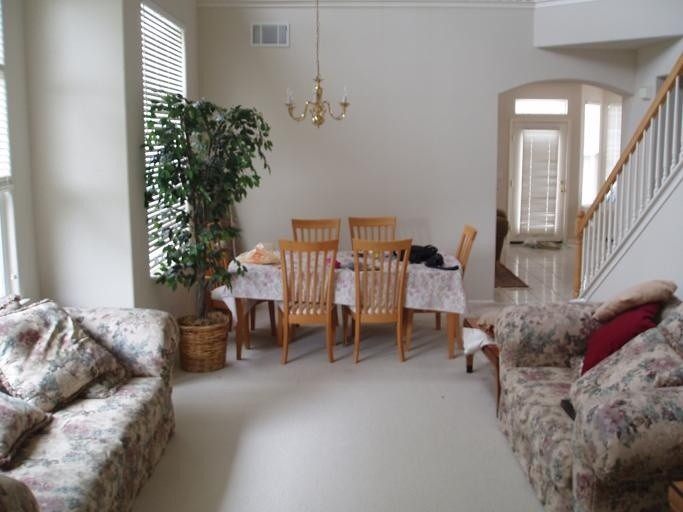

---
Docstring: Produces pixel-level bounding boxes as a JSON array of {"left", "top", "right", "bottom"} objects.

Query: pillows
[
  {"left": 590, "top": 278, "right": 677, "bottom": 327},
  {"left": 0, "top": 296, "right": 132, "bottom": 411},
  {"left": 565, "top": 327, "right": 683, "bottom": 424},
  {"left": 658, "top": 298, "right": 683, "bottom": 360},
  {"left": 0, "top": 390, "right": 53, "bottom": 470},
  {"left": 575, "top": 301, "right": 667, "bottom": 377}
]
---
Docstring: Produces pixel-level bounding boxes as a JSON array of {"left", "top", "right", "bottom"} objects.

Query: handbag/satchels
[{"left": 400, "top": 246, "right": 437, "bottom": 263}]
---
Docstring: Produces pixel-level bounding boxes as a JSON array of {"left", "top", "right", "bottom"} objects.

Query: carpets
[{"left": 495, "top": 256, "right": 529, "bottom": 291}]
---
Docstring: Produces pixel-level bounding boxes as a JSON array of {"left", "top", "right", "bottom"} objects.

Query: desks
[
  {"left": 226, "top": 249, "right": 467, "bottom": 364},
  {"left": 461, "top": 314, "right": 502, "bottom": 418}
]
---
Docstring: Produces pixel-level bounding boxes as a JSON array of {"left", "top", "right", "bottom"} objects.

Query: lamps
[{"left": 284, "top": 1, "right": 351, "bottom": 131}]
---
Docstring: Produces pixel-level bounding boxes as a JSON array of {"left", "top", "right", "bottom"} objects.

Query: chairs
[
  {"left": 400, "top": 223, "right": 476, "bottom": 360},
  {"left": 269, "top": 238, "right": 341, "bottom": 364},
  {"left": 286, "top": 216, "right": 341, "bottom": 245},
  {"left": 337, "top": 235, "right": 419, "bottom": 364},
  {"left": 198, "top": 219, "right": 276, "bottom": 353},
  {"left": 344, "top": 213, "right": 397, "bottom": 245}
]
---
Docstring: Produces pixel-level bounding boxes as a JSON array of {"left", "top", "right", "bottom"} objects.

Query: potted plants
[{"left": 128, "top": 89, "right": 270, "bottom": 380}]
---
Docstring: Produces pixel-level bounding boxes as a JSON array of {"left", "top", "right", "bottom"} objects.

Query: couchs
[
  {"left": 494, "top": 285, "right": 682, "bottom": 511},
  {"left": 0, "top": 296, "right": 180, "bottom": 512}
]
